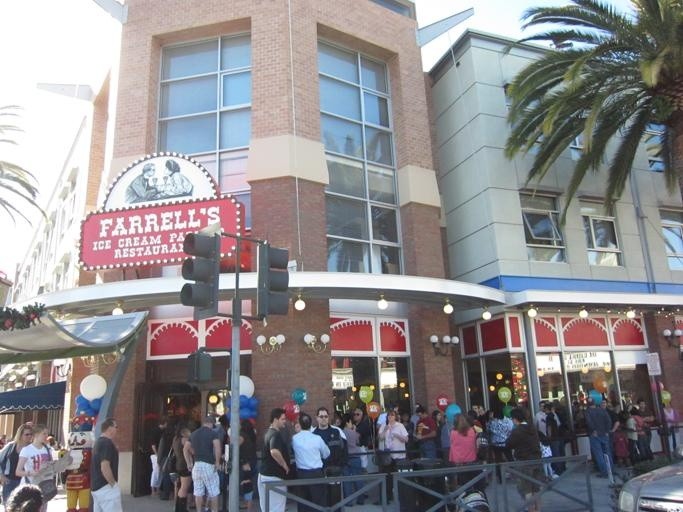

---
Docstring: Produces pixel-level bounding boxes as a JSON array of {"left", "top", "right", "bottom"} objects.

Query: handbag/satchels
[
  {"left": 37, "top": 479, "right": 57, "bottom": 504},
  {"left": 372, "top": 450, "right": 391, "bottom": 466},
  {"left": 162, "top": 456, "right": 176, "bottom": 473}
]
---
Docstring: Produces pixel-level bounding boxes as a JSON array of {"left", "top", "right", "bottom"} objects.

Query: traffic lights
[
  {"left": 179, "top": 232, "right": 220, "bottom": 323},
  {"left": 257, "top": 241, "right": 289, "bottom": 321}
]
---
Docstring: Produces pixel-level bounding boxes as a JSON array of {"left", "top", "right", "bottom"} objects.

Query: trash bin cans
[{"left": 396, "top": 458, "right": 446, "bottom": 512}]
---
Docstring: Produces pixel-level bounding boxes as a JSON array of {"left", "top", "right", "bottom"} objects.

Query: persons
[
  {"left": 257, "top": 407, "right": 290, "bottom": 512},
  {"left": 125, "top": 160, "right": 162, "bottom": 204},
  {"left": 571, "top": 392, "right": 655, "bottom": 479},
  {"left": 0, "top": 422, "right": 60, "bottom": 512},
  {"left": 161, "top": 159, "right": 194, "bottom": 199},
  {"left": 291, "top": 406, "right": 369, "bottom": 512},
  {"left": 58, "top": 412, "right": 94, "bottom": 512},
  {"left": 89, "top": 419, "right": 122, "bottom": 512},
  {"left": 148, "top": 413, "right": 257, "bottom": 512},
  {"left": 508, "top": 409, "right": 542, "bottom": 512},
  {"left": 376, "top": 397, "right": 567, "bottom": 488}
]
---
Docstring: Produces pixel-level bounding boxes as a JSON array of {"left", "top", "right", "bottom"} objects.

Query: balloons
[
  {"left": 587, "top": 374, "right": 608, "bottom": 408},
  {"left": 283, "top": 387, "right": 307, "bottom": 422},
  {"left": 359, "top": 386, "right": 373, "bottom": 403},
  {"left": 498, "top": 386, "right": 514, "bottom": 404},
  {"left": 436, "top": 393, "right": 463, "bottom": 426},
  {"left": 74, "top": 374, "right": 107, "bottom": 417},
  {"left": 225, "top": 374, "right": 259, "bottom": 435},
  {"left": 651, "top": 381, "right": 672, "bottom": 406},
  {"left": 366, "top": 402, "right": 380, "bottom": 419}
]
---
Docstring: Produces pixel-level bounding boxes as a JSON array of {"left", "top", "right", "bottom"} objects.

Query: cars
[{"left": 617, "top": 461, "right": 682, "bottom": 511}]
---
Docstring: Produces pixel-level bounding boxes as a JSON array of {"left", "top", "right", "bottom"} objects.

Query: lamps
[
  {"left": 441, "top": 297, "right": 454, "bottom": 315},
  {"left": 429, "top": 333, "right": 459, "bottom": 357},
  {"left": 303, "top": 332, "right": 330, "bottom": 354},
  {"left": 111, "top": 299, "right": 125, "bottom": 318},
  {"left": 256, "top": 334, "right": 285, "bottom": 355},
  {"left": 378, "top": 292, "right": 389, "bottom": 310},
  {"left": 478, "top": 300, "right": 683, "bottom": 353},
  {"left": 295, "top": 294, "right": 306, "bottom": 311}
]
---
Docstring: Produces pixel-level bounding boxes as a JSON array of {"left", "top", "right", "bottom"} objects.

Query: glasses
[
  {"left": 354, "top": 413, "right": 360, "bottom": 416},
  {"left": 317, "top": 415, "right": 328, "bottom": 418}
]
